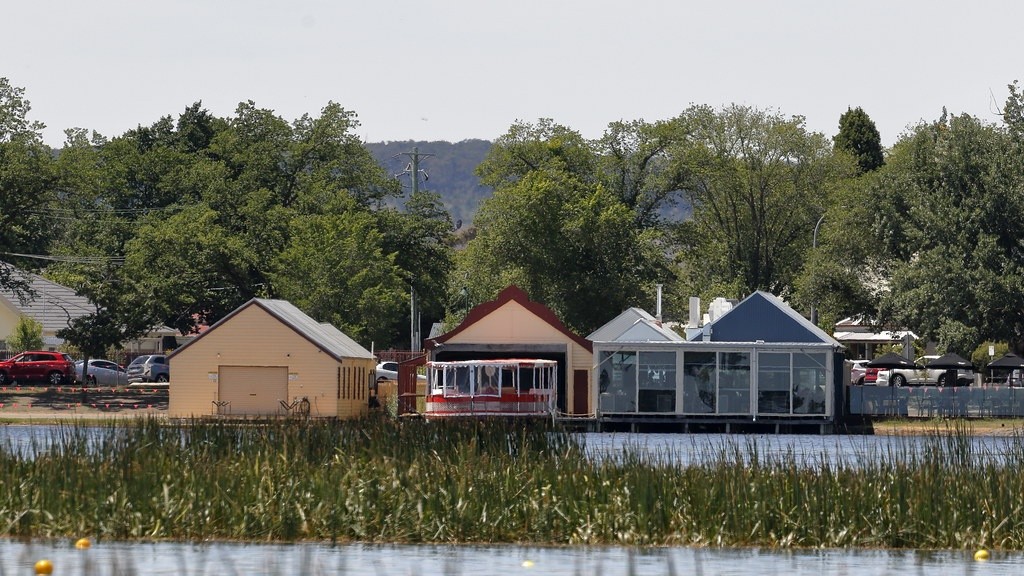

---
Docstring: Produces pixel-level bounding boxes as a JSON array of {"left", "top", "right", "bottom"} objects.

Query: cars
[
  {"left": 845, "top": 355, "right": 1024, "bottom": 388},
  {"left": 376, "top": 361, "right": 426, "bottom": 381},
  {"left": 74, "top": 358, "right": 128, "bottom": 387}
]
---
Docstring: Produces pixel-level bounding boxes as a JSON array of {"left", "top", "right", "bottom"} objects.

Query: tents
[{"left": 424, "top": 358, "right": 558, "bottom": 416}]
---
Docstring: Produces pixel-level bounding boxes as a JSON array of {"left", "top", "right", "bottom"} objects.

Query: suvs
[
  {"left": 0, "top": 350, "right": 76, "bottom": 386},
  {"left": 127, "top": 354, "right": 170, "bottom": 383}
]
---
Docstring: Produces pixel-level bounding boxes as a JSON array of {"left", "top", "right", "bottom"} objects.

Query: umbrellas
[
  {"left": 985, "top": 351, "right": 1024, "bottom": 387},
  {"left": 864, "top": 350, "right": 926, "bottom": 386},
  {"left": 925, "top": 352, "right": 979, "bottom": 386}
]
